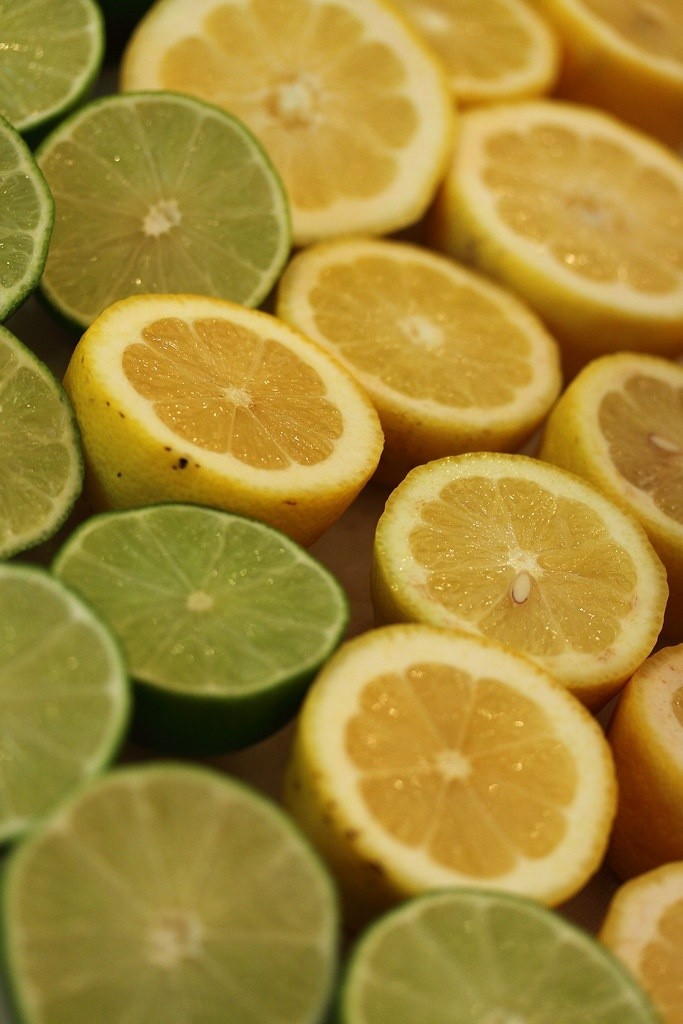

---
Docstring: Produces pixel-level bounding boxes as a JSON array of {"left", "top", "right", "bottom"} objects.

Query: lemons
[{"left": 0, "top": 0, "right": 683, "bottom": 1024}]
